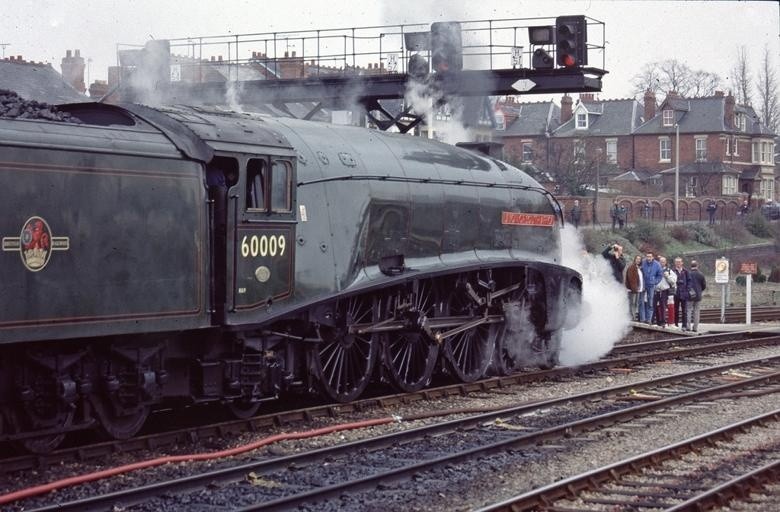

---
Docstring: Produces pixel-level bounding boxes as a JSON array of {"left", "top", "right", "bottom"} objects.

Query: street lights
[{"left": 673, "top": 124, "right": 680, "bottom": 222}]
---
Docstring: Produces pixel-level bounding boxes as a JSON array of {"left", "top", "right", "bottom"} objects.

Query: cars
[{"left": 736, "top": 200, "right": 780, "bottom": 223}]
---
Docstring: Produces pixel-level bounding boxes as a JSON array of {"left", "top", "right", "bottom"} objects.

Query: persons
[
  {"left": 213, "top": 166, "right": 238, "bottom": 258},
  {"left": 602, "top": 244, "right": 706, "bottom": 331},
  {"left": 706, "top": 196, "right": 778, "bottom": 227},
  {"left": 612, "top": 201, "right": 650, "bottom": 231},
  {"left": 554, "top": 197, "right": 595, "bottom": 229}
]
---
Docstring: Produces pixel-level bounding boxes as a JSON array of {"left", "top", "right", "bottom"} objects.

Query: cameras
[{"left": 614, "top": 246, "right": 618, "bottom": 251}]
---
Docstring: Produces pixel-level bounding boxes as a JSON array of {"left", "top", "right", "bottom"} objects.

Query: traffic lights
[
  {"left": 430, "top": 22, "right": 463, "bottom": 73},
  {"left": 555, "top": 15, "right": 588, "bottom": 70}
]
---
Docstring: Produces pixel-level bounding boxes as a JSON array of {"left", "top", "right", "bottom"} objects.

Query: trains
[{"left": 1, "top": 101, "right": 586, "bottom": 457}]
